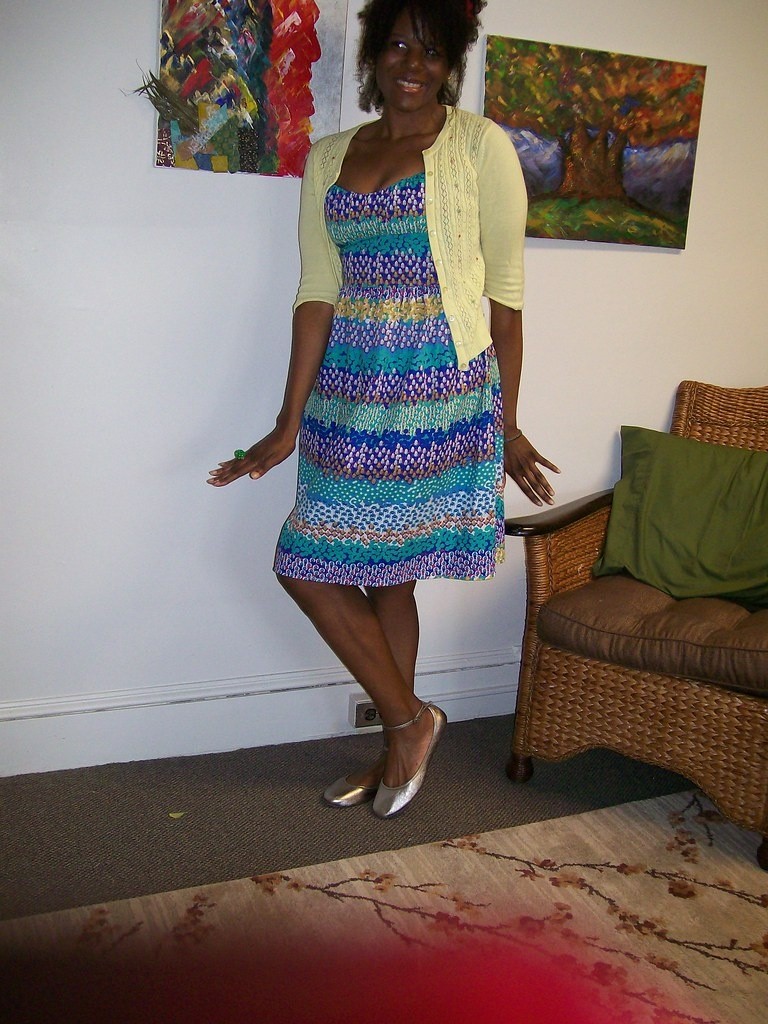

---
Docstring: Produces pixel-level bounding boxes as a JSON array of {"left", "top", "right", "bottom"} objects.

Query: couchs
[{"left": 500, "top": 382, "right": 768, "bottom": 873}]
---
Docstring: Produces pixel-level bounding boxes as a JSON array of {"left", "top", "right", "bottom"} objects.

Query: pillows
[{"left": 591, "top": 426, "right": 767, "bottom": 601}]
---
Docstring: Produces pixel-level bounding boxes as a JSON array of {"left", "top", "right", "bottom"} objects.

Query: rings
[{"left": 233, "top": 450, "right": 247, "bottom": 463}]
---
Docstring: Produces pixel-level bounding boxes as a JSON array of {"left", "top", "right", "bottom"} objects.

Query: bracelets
[{"left": 504, "top": 430, "right": 522, "bottom": 448}]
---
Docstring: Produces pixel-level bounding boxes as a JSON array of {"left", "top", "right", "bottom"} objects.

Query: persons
[{"left": 205, "top": 0, "right": 561, "bottom": 820}]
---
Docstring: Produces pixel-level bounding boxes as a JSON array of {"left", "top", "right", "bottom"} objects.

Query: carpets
[{"left": 1, "top": 790, "right": 768, "bottom": 1024}]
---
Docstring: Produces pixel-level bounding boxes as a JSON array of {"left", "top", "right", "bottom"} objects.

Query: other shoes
[
  {"left": 372, "top": 700, "right": 447, "bottom": 820},
  {"left": 323, "top": 744, "right": 388, "bottom": 808}
]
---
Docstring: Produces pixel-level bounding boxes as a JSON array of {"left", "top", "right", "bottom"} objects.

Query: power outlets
[{"left": 348, "top": 694, "right": 382, "bottom": 728}]
